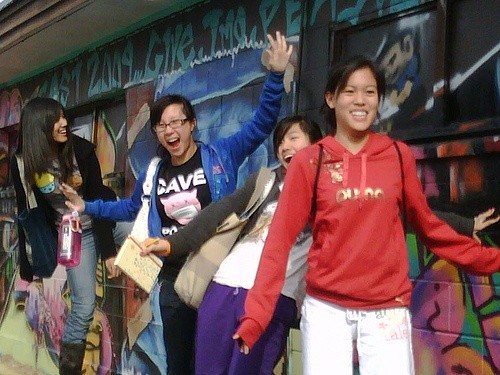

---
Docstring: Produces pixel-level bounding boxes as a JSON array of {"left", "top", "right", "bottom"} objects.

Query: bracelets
[{"left": 153, "top": 239, "right": 171, "bottom": 258}]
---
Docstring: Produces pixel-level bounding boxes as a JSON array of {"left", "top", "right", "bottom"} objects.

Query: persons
[
  {"left": 58, "top": 31, "right": 293, "bottom": 375},
  {"left": 10, "top": 97, "right": 134, "bottom": 375},
  {"left": 231, "top": 55, "right": 500, "bottom": 375},
  {"left": 136, "top": 114, "right": 500, "bottom": 375}
]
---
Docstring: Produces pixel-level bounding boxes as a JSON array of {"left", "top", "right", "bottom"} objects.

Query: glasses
[{"left": 151, "top": 118, "right": 188, "bottom": 133}]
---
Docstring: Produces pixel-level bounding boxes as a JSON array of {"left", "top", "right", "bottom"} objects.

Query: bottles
[{"left": 57, "top": 213, "right": 82, "bottom": 267}]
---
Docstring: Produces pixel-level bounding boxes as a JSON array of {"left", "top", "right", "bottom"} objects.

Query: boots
[{"left": 59, "top": 342, "right": 86, "bottom": 375}]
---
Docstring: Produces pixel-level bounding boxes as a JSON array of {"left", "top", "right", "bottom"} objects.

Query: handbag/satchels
[
  {"left": 132, "top": 157, "right": 163, "bottom": 242},
  {"left": 174, "top": 167, "right": 270, "bottom": 307},
  {"left": 16, "top": 153, "right": 58, "bottom": 277}
]
